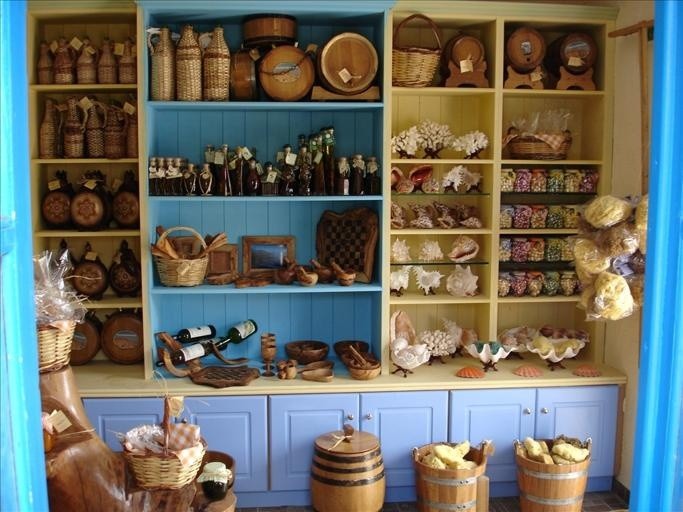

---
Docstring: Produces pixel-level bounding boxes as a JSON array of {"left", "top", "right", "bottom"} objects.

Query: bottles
[
  {"left": 148, "top": 126, "right": 379, "bottom": 195},
  {"left": 154, "top": 319, "right": 258, "bottom": 367}
]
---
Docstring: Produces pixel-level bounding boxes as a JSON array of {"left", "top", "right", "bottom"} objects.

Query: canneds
[{"left": 497, "top": 169, "right": 597, "bottom": 298}]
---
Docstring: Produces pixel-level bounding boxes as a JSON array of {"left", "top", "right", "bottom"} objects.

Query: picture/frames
[{"left": 242, "top": 235, "right": 296, "bottom": 279}]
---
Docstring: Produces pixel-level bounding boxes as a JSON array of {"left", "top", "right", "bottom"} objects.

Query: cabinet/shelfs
[{"left": 24, "top": 1, "right": 629, "bottom": 506}]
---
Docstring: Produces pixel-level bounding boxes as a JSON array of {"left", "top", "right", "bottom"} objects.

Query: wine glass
[{"left": 261, "top": 333, "right": 277, "bottom": 376}]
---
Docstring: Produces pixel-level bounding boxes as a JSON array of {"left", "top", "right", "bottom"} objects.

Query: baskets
[
  {"left": 154, "top": 225, "right": 209, "bottom": 287},
  {"left": 392, "top": 13, "right": 443, "bottom": 87},
  {"left": 507, "top": 126, "right": 572, "bottom": 159},
  {"left": 36, "top": 318, "right": 77, "bottom": 373},
  {"left": 122, "top": 397, "right": 209, "bottom": 490}
]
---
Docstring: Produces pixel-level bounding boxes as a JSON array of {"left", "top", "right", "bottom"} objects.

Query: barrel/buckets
[
  {"left": 412, "top": 442, "right": 495, "bottom": 512},
  {"left": 311, "top": 424, "right": 386, "bottom": 511},
  {"left": 516, "top": 437, "right": 590, "bottom": 512}
]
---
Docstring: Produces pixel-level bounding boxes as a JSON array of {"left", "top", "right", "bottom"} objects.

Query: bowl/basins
[
  {"left": 230, "top": 15, "right": 379, "bottom": 102},
  {"left": 285, "top": 340, "right": 329, "bottom": 364},
  {"left": 334, "top": 340, "right": 381, "bottom": 380}
]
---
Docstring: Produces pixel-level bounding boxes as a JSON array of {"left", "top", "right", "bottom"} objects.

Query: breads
[
  {"left": 502, "top": 327, "right": 581, "bottom": 356},
  {"left": 421, "top": 441, "right": 479, "bottom": 471},
  {"left": 514, "top": 434, "right": 590, "bottom": 465},
  {"left": 572, "top": 193, "right": 648, "bottom": 321}
]
